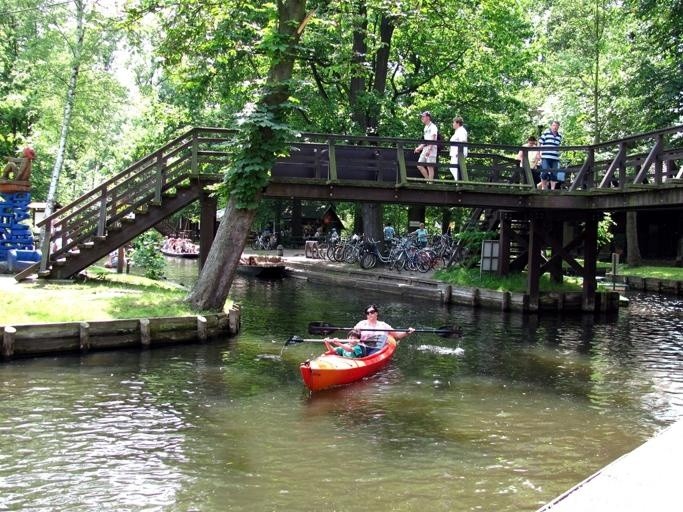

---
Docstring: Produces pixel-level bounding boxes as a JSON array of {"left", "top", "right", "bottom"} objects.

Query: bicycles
[
  {"left": 251, "top": 231, "right": 272, "bottom": 250},
  {"left": 326, "top": 226, "right": 456, "bottom": 273}
]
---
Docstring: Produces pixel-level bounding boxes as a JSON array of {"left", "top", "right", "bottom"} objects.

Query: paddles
[
  {"left": 308, "top": 321, "right": 463, "bottom": 340},
  {"left": 284, "top": 334, "right": 388, "bottom": 346}
]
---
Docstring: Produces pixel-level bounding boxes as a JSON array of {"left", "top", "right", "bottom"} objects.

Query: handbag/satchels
[{"left": 437, "top": 133, "right": 445, "bottom": 150}]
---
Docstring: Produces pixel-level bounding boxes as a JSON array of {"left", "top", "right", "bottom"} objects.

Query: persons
[
  {"left": 449, "top": 116, "right": 468, "bottom": 187},
  {"left": 323, "top": 329, "right": 363, "bottom": 358},
  {"left": 53, "top": 230, "right": 77, "bottom": 260},
  {"left": 161, "top": 234, "right": 197, "bottom": 255},
  {"left": 414, "top": 111, "right": 438, "bottom": 184},
  {"left": 383, "top": 222, "right": 395, "bottom": 243},
  {"left": 354, "top": 304, "right": 417, "bottom": 356},
  {"left": 430, "top": 132, "right": 445, "bottom": 179},
  {"left": 304, "top": 225, "right": 339, "bottom": 248},
  {"left": 411, "top": 222, "right": 431, "bottom": 250},
  {"left": 518, "top": 136, "right": 538, "bottom": 191},
  {"left": 535, "top": 120, "right": 562, "bottom": 191},
  {"left": 239, "top": 255, "right": 284, "bottom": 266},
  {"left": 105, "top": 243, "right": 137, "bottom": 270}
]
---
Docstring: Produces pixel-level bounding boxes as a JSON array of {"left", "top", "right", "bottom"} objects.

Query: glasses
[{"left": 365, "top": 310, "right": 376, "bottom": 315}]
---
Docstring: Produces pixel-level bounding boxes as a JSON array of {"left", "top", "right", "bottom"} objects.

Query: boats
[
  {"left": 160, "top": 237, "right": 200, "bottom": 256},
  {"left": 299, "top": 334, "right": 397, "bottom": 393},
  {"left": 237, "top": 253, "right": 288, "bottom": 276}
]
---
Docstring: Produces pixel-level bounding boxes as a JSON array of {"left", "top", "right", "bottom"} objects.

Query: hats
[{"left": 419, "top": 111, "right": 431, "bottom": 117}]
